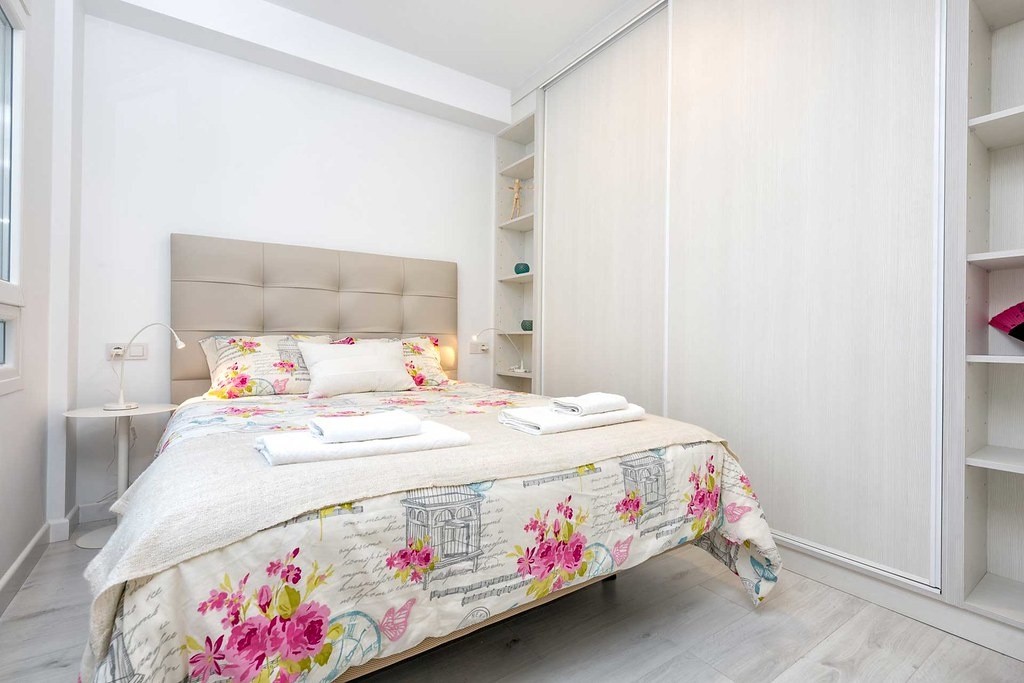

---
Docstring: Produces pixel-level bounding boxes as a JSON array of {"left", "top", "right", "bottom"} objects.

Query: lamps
[
  {"left": 102, "top": 322, "right": 186, "bottom": 411},
  {"left": 471, "top": 327, "right": 530, "bottom": 373}
]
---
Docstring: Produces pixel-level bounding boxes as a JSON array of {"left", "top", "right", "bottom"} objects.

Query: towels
[
  {"left": 255, "top": 409, "right": 471, "bottom": 466},
  {"left": 498, "top": 392, "right": 646, "bottom": 435}
]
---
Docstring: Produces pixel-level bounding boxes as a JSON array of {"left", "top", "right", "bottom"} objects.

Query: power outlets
[
  {"left": 105, "top": 342, "right": 148, "bottom": 361},
  {"left": 468, "top": 341, "right": 490, "bottom": 354}
]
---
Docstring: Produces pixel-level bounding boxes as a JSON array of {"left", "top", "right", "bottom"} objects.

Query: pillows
[
  {"left": 330, "top": 335, "right": 458, "bottom": 386},
  {"left": 198, "top": 334, "right": 336, "bottom": 400},
  {"left": 298, "top": 341, "right": 420, "bottom": 400}
]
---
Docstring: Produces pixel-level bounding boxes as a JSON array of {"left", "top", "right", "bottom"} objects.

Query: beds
[{"left": 76, "top": 232, "right": 783, "bottom": 683}]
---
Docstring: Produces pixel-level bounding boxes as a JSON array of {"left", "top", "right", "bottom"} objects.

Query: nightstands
[{"left": 62, "top": 402, "right": 180, "bottom": 549}]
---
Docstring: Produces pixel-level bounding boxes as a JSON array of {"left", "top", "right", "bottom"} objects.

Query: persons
[{"left": 510, "top": 178, "right": 523, "bottom": 219}]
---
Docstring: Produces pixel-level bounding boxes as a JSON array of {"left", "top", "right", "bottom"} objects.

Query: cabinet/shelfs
[
  {"left": 492, "top": 111, "right": 538, "bottom": 393},
  {"left": 942, "top": 0, "right": 1024, "bottom": 633},
  {"left": 532, "top": 0, "right": 950, "bottom": 629}
]
